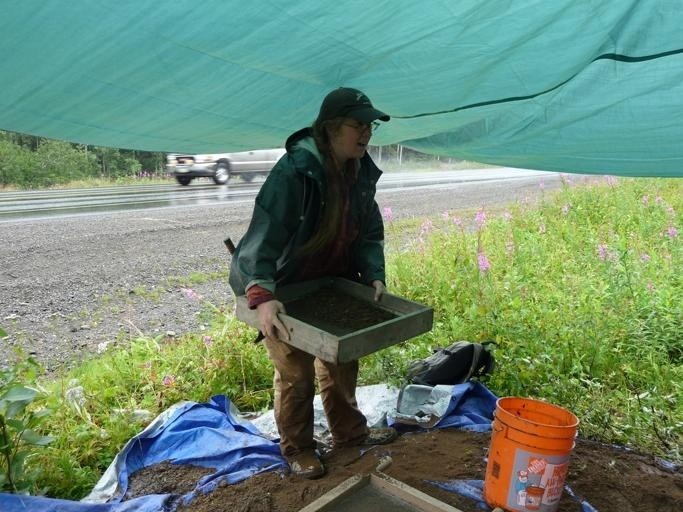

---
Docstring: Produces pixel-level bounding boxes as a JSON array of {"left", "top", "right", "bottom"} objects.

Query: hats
[{"left": 321, "top": 88, "right": 390, "bottom": 121}]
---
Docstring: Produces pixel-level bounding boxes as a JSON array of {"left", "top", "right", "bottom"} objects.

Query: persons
[{"left": 228, "top": 86, "right": 397, "bottom": 479}]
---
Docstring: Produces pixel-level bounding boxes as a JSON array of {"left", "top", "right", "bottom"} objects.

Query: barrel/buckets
[{"left": 482, "top": 396, "right": 579, "bottom": 512}]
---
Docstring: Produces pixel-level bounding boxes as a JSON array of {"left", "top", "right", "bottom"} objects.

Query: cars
[{"left": 166, "top": 148, "right": 288, "bottom": 185}]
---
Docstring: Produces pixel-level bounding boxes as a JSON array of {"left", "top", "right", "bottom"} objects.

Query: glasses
[{"left": 343, "top": 122, "right": 380, "bottom": 130}]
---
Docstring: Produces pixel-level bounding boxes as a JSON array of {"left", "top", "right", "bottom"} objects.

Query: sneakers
[
  {"left": 288, "top": 449, "right": 324, "bottom": 479},
  {"left": 332, "top": 427, "right": 396, "bottom": 447}
]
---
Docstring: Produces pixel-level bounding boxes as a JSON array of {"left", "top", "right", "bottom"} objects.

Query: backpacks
[{"left": 407, "top": 341, "right": 483, "bottom": 386}]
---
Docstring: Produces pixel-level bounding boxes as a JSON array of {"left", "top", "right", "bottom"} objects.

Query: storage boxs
[
  {"left": 299, "top": 456, "right": 463, "bottom": 512},
  {"left": 235, "top": 274, "right": 434, "bottom": 366}
]
